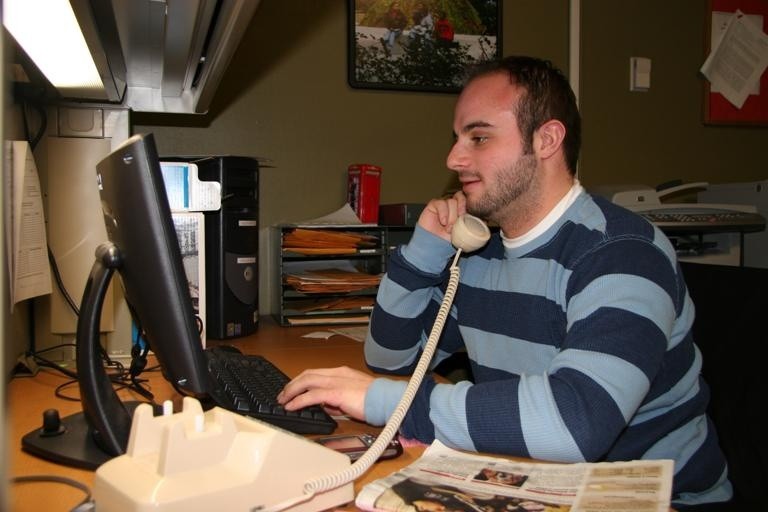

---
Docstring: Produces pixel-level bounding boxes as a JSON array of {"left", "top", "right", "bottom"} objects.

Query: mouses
[{"left": 207, "top": 344, "right": 243, "bottom": 354}]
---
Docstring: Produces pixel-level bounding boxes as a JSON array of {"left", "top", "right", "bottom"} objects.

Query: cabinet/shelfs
[{"left": 263, "top": 221, "right": 415, "bottom": 329}]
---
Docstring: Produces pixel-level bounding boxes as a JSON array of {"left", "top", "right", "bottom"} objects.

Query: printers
[{"left": 592, "top": 177, "right": 766, "bottom": 266}]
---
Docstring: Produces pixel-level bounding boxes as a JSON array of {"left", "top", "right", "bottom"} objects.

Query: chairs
[{"left": 678, "top": 259, "right": 764, "bottom": 512}]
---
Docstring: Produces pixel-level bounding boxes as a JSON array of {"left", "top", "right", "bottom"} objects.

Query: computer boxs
[{"left": 158, "top": 155, "right": 259, "bottom": 339}]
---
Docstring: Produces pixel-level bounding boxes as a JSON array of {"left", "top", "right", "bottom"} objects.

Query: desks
[{"left": 5, "top": 317, "right": 672, "bottom": 512}]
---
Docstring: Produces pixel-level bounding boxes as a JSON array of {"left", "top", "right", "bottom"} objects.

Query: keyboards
[{"left": 205, "top": 353, "right": 338, "bottom": 435}]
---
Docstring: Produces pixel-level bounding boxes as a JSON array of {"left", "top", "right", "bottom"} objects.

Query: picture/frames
[{"left": 346, "top": 0, "right": 503, "bottom": 95}]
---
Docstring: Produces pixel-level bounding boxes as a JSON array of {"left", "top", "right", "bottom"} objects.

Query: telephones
[{"left": 451, "top": 214, "right": 492, "bottom": 253}]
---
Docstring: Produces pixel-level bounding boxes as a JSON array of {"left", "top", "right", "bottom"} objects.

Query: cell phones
[{"left": 313, "top": 432, "right": 403, "bottom": 464}]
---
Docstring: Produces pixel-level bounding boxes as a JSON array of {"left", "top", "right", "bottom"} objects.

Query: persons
[
  {"left": 380, "top": 2, "right": 456, "bottom": 53},
  {"left": 276, "top": 53, "right": 726, "bottom": 512}
]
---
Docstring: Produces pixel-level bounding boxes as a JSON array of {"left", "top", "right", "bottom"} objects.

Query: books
[
  {"left": 700, "top": 8, "right": 768, "bottom": 109},
  {"left": 282, "top": 202, "right": 383, "bottom": 325},
  {"left": 355, "top": 439, "right": 675, "bottom": 512}
]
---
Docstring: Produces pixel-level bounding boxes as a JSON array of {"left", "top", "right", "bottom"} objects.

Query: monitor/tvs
[{"left": 21, "top": 132, "right": 215, "bottom": 470}]
